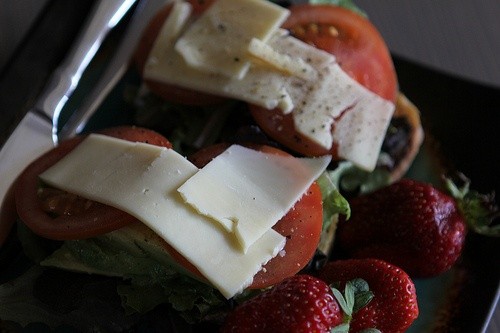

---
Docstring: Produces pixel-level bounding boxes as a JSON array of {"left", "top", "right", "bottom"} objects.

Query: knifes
[{"left": 0, "top": 0, "right": 138, "bottom": 208}]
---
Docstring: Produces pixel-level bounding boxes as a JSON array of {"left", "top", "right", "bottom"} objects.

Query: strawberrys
[{"left": 218, "top": 178, "right": 467, "bottom": 333}]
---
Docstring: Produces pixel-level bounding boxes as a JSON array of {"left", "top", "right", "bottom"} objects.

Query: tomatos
[{"left": 12, "top": 0, "right": 399, "bottom": 290}]
